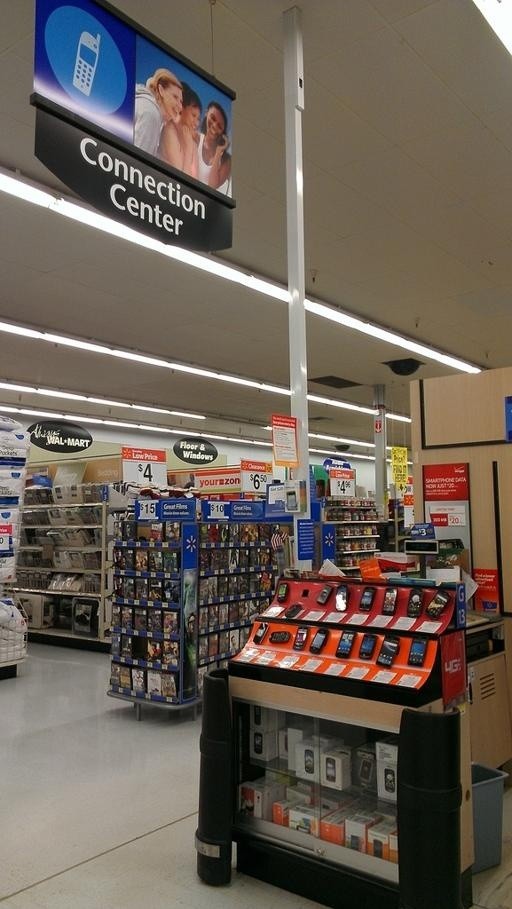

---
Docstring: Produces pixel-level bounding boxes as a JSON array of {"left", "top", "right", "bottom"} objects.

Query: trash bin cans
[{"left": 471, "top": 761, "right": 509, "bottom": 876}]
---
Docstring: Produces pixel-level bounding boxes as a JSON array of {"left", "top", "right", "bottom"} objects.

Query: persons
[
  {"left": 158, "top": 81, "right": 203, "bottom": 178},
  {"left": 194, "top": 100, "right": 231, "bottom": 198},
  {"left": 133, "top": 66, "right": 184, "bottom": 157}
]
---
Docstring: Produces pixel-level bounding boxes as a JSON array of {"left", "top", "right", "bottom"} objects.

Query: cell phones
[
  {"left": 335, "top": 630, "right": 356, "bottom": 658},
  {"left": 316, "top": 584, "right": 333, "bottom": 605},
  {"left": 285, "top": 604, "right": 302, "bottom": 618},
  {"left": 253, "top": 622, "right": 270, "bottom": 644},
  {"left": 250, "top": 705, "right": 397, "bottom": 862},
  {"left": 335, "top": 584, "right": 347, "bottom": 612},
  {"left": 358, "top": 633, "right": 376, "bottom": 659},
  {"left": 309, "top": 628, "right": 329, "bottom": 654},
  {"left": 425, "top": 589, "right": 451, "bottom": 619},
  {"left": 376, "top": 633, "right": 401, "bottom": 667},
  {"left": 270, "top": 631, "right": 290, "bottom": 642},
  {"left": 359, "top": 587, "right": 376, "bottom": 611},
  {"left": 382, "top": 588, "right": 398, "bottom": 616},
  {"left": 407, "top": 636, "right": 428, "bottom": 666},
  {"left": 277, "top": 583, "right": 288, "bottom": 602},
  {"left": 407, "top": 588, "right": 422, "bottom": 617},
  {"left": 293, "top": 627, "right": 308, "bottom": 650}
]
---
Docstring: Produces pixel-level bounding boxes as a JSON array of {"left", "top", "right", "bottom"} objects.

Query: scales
[{"left": 404, "top": 540, "right": 439, "bottom": 579}]
